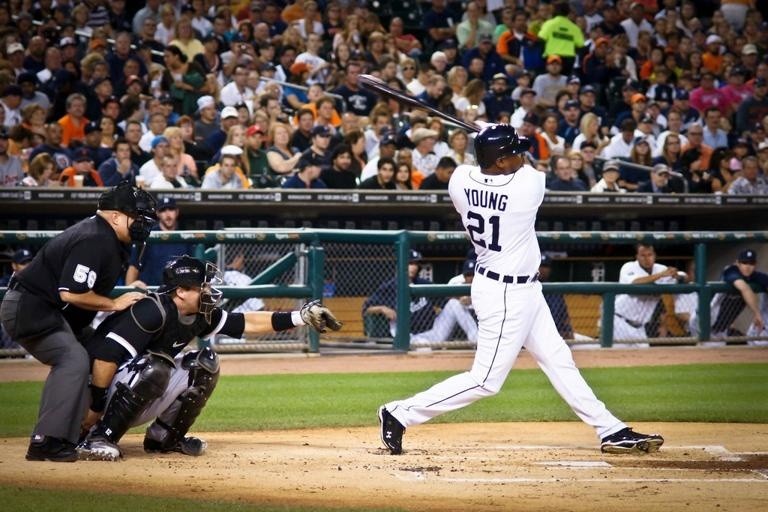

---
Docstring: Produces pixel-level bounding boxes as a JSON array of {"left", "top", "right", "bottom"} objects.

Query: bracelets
[
  {"left": 90, "top": 383, "right": 108, "bottom": 414},
  {"left": 270, "top": 311, "right": 310, "bottom": 333}
]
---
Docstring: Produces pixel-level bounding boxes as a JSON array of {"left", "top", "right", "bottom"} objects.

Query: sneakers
[
  {"left": 377, "top": 405, "right": 403, "bottom": 457},
  {"left": 144, "top": 428, "right": 207, "bottom": 456},
  {"left": 25, "top": 433, "right": 78, "bottom": 463},
  {"left": 600, "top": 427, "right": 665, "bottom": 455},
  {"left": 76, "top": 427, "right": 122, "bottom": 460}
]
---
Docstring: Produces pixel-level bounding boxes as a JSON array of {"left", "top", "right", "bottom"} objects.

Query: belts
[{"left": 614, "top": 313, "right": 645, "bottom": 329}]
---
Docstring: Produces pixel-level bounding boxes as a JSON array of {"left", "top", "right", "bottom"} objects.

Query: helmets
[
  {"left": 474, "top": 123, "right": 531, "bottom": 172},
  {"left": 4, "top": 4, "right": 250, "bottom": 165},
  {"left": 98, "top": 184, "right": 160, "bottom": 246},
  {"left": 154, "top": 254, "right": 224, "bottom": 325}
]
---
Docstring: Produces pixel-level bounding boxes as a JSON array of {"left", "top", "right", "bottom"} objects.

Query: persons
[
  {"left": 77, "top": 255, "right": 343, "bottom": 462},
  {"left": 379, "top": 121, "right": 663, "bottom": 456},
  {"left": 1, "top": 182, "right": 156, "bottom": 461}
]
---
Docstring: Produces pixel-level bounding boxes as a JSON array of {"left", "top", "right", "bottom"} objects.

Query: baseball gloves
[{"left": 302, "top": 299, "right": 343, "bottom": 336}]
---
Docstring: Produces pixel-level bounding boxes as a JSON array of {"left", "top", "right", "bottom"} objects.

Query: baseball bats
[{"left": 356, "top": 72, "right": 480, "bottom": 133}]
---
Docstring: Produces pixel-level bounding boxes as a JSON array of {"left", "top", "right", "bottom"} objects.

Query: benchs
[{"left": 245, "top": 289, "right": 686, "bottom": 342}]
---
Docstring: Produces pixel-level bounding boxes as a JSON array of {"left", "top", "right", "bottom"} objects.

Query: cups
[{"left": 72, "top": 175, "right": 85, "bottom": 188}]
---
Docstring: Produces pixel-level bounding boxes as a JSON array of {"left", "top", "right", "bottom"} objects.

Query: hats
[
  {"left": 410, "top": 127, "right": 438, "bottom": 143},
  {"left": 289, "top": 61, "right": 317, "bottom": 78},
  {"left": 736, "top": 249, "right": 757, "bottom": 266}
]
